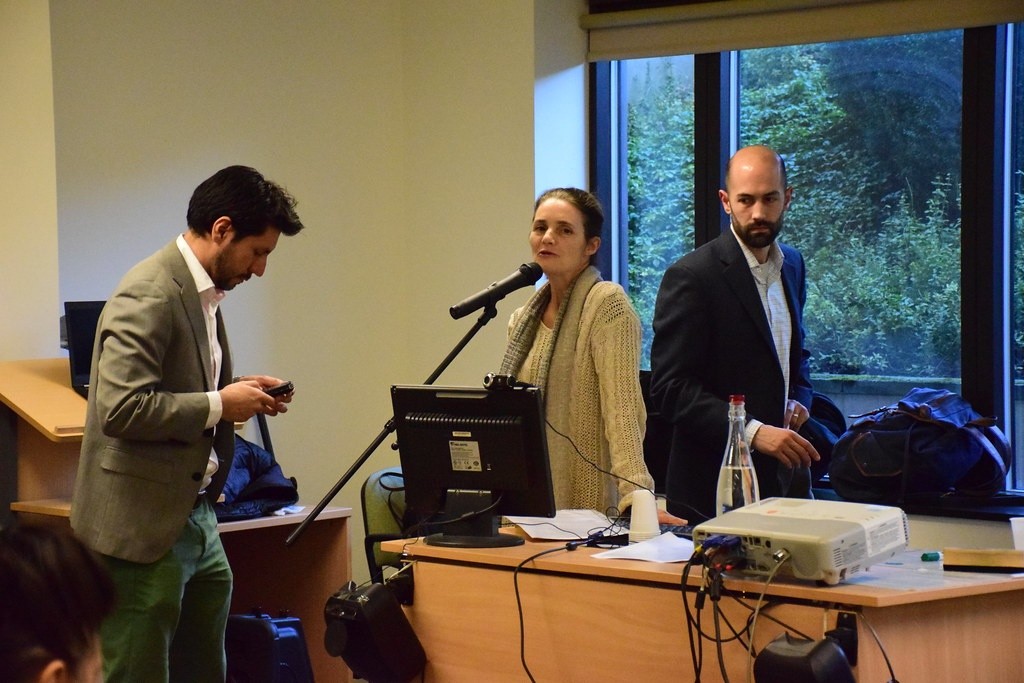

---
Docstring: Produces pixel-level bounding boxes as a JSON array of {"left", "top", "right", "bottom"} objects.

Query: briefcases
[{"left": 221, "top": 611, "right": 318, "bottom": 683}]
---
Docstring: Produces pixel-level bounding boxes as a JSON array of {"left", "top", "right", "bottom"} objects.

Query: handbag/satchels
[
  {"left": 796, "top": 389, "right": 848, "bottom": 487},
  {"left": 825, "top": 385, "right": 1015, "bottom": 518}
]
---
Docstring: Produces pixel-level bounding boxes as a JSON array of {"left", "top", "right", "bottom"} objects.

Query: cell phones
[{"left": 263, "top": 381, "right": 294, "bottom": 397}]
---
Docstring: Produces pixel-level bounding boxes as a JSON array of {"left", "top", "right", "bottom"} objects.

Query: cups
[
  {"left": 1009, "top": 517, "right": 1024, "bottom": 550},
  {"left": 628, "top": 489, "right": 661, "bottom": 545}
]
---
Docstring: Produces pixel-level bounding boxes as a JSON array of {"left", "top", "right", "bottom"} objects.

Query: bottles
[{"left": 715, "top": 395, "right": 760, "bottom": 519}]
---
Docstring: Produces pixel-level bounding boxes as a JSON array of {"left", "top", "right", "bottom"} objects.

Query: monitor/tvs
[{"left": 390, "top": 385, "right": 556, "bottom": 548}]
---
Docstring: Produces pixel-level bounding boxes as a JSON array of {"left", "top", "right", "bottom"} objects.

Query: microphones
[{"left": 449, "top": 262, "right": 542, "bottom": 320}]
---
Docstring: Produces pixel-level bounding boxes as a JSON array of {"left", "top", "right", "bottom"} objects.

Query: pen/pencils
[{"left": 919, "top": 550, "right": 942, "bottom": 563}]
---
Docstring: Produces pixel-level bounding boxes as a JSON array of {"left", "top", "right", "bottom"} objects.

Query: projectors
[{"left": 692, "top": 497, "right": 909, "bottom": 585}]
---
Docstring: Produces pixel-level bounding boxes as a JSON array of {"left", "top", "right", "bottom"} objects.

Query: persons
[
  {"left": 68, "top": 165, "right": 305, "bottom": 683},
  {"left": 648, "top": 145, "right": 821, "bottom": 525},
  {"left": 0, "top": 515, "right": 121, "bottom": 683},
  {"left": 499, "top": 188, "right": 688, "bottom": 527}
]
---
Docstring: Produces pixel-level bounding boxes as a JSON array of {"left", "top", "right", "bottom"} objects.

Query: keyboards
[{"left": 620, "top": 520, "right": 694, "bottom": 540}]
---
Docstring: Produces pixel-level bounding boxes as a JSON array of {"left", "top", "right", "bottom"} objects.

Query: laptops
[{"left": 64, "top": 300, "right": 107, "bottom": 398}]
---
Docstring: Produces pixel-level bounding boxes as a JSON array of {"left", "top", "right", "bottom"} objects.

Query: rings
[{"left": 793, "top": 413, "right": 799, "bottom": 418}]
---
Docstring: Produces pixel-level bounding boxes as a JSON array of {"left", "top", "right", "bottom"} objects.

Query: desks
[
  {"left": 0, "top": 357, "right": 355, "bottom": 683},
  {"left": 380, "top": 527, "right": 1024, "bottom": 683}
]
